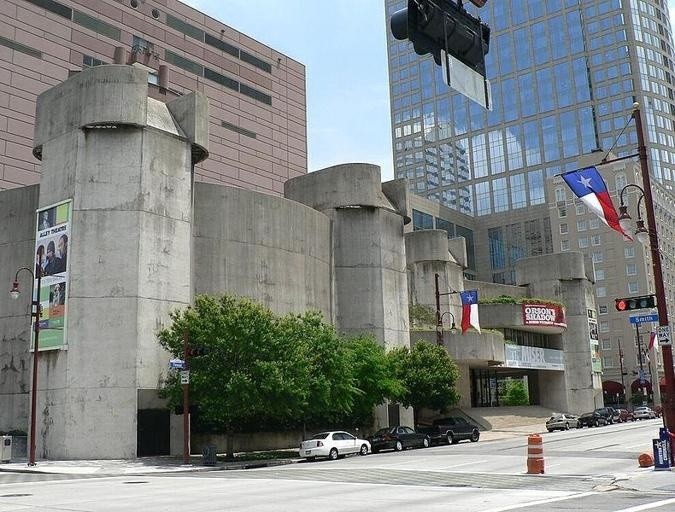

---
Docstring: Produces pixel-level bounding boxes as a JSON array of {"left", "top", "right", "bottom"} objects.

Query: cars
[
  {"left": 299, "top": 417, "right": 479, "bottom": 460},
  {"left": 546, "top": 406, "right": 662, "bottom": 432}
]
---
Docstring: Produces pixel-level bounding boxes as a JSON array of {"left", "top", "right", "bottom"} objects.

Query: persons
[
  {"left": 35, "top": 245, "right": 44, "bottom": 278},
  {"left": 44, "top": 240, "right": 62, "bottom": 275},
  {"left": 56, "top": 234, "right": 66, "bottom": 271},
  {"left": 37, "top": 211, "right": 52, "bottom": 231}
]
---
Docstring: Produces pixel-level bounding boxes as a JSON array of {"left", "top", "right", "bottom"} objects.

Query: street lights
[
  {"left": 10, "top": 247, "right": 43, "bottom": 466},
  {"left": 553, "top": 102, "right": 675, "bottom": 466},
  {"left": 435, "top": 273, "right": 480, "bottom": 348}
]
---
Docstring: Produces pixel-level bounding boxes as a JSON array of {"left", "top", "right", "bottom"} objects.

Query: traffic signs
[{"left": 656, "top": 325, "right": 672, "bottom": 346}]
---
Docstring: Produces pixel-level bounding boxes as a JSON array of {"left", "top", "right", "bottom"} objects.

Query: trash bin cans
[
  {"left": 0, "top": 435, "right": 12, "bottom": 464},
  {"left": 202, "top": 443, "right": 217, "bottom": 466}
]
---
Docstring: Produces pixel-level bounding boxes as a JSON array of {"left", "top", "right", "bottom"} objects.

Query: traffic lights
[
  {"left": 390, "top": 0, "right": 493, "bottom": 110},
  {"left": 188, "top": 346, "right": 212, "bottom": 357},
  {"left": 614, "top": 294, "right": 656, "bottom": 311}
]
